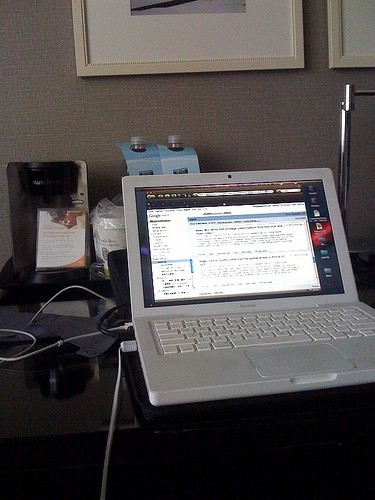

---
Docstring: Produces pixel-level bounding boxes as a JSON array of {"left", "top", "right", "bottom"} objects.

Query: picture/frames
[
  {"left": 72, "top": 0, "right": 305, "bottom": 76},
  {"left": 327, "top": 0, "right": 375, "bottom": 70}
]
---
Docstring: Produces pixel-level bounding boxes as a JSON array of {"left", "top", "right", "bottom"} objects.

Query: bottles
[
  {"left": 119, "top": 136, "right": 167, "bottom": 176},
  {"left": 158, "top": 135, "right": 199, "bottom": 175}
]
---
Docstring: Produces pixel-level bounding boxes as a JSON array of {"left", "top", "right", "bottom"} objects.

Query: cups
[{"left": 94, "top": 208, "right": 130, "bottom": 279}]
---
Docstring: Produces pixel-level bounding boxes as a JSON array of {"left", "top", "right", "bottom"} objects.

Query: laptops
[{"left": 122, "top": 168, "right": 375, "bottom": 406}]
